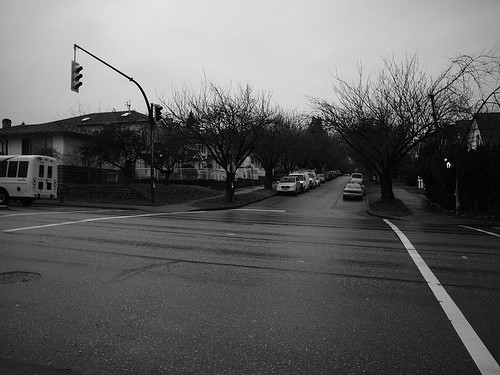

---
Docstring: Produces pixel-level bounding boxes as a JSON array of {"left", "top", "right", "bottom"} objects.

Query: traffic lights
[
  {"left": 156, "top": 105, "right": 163, "bottom": 122},
  {"left": 70, "top": 60, "right": 83, "bottom": 93}
]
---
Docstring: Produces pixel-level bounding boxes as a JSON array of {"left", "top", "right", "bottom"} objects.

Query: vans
[{"left": 0, "top": 155, "right": 58, "bottom": 205}]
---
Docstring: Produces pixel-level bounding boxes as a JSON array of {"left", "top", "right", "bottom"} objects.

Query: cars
[{"left": 277, "top": 170, "right": 364, "bottom": 201}]
